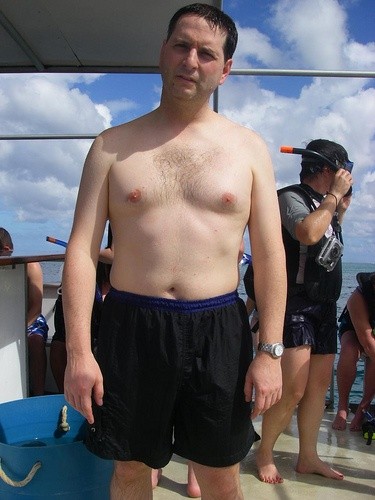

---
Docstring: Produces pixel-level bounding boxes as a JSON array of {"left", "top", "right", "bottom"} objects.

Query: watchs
[{"left": 258, "top": 343, "right": 285, "bottom": 359}]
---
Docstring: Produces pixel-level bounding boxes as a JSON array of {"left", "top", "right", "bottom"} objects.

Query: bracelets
[{"left": 325, "top": 190, "right": 338, "bottom": 213}]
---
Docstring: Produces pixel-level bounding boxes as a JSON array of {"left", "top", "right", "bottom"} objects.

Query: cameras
[{"left": 315, "top": 236, "right": 344, "bottom": 273}]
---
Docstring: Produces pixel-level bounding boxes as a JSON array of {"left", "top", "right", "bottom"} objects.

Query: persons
[
  {"left": 243, "top": 139, "right": 354, "bottom": 484},
  {"left": 0, "top": 228, "right": 113, "bottom": 396},
  {"left": 61, "top": 3, "right": 288, "bottom": 500},
  {"left": 331, "top": 272, "right": 375, "bottom": 431}
]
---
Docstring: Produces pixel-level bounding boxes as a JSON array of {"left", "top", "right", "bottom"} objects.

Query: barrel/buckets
[{"left": 0, "top": 394, "right": 115, "bottom": 500}]
